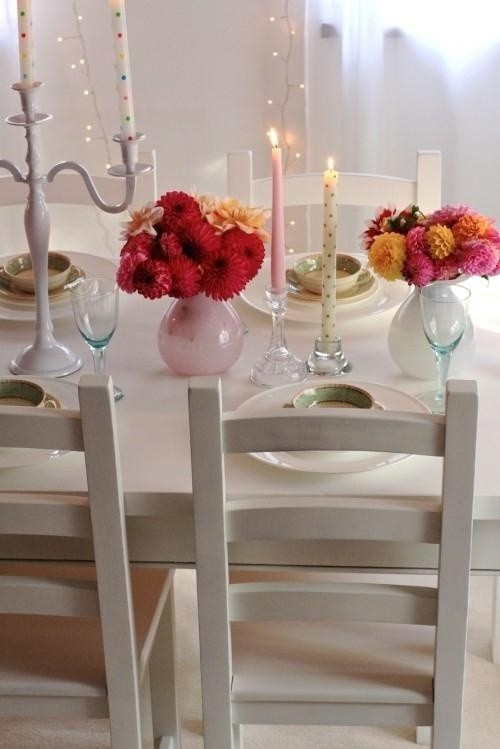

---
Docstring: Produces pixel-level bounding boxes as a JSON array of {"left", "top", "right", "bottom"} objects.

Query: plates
[
  {"left": 238, "top": 251, "right": 416, "bottom": 325},
  {"left": 0, "top": 374, "right": 81, "bottom": 469},
  {"left": 233, "top": 379, "right": 435, "bottom": 475},
  {"left": 0, "top": 251, "right": 125, "bottom": 322}
]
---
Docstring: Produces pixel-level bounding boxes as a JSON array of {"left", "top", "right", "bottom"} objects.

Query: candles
[
  {"left": 271, "top": 148, "right": 291, "bottom": 293},
  {"left": 18, "top": 2, "right": 37, "bottom": 88},
  {"left": 321, "top": 173, "right": 338, "bottom": 351},
  {"left": 108, "top": 1, "right": 137, "bottom": 142}
]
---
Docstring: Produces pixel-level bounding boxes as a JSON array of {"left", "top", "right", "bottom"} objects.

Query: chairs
[
  {"left": 1, "top": 373, "right": 177, "bottom": 749},
  {"left": 226, "top": 146, "right": 444, "bottom": 254},
  {"left": 167, "top": 374, "right": 499, "bottom": 748},
  {"left": 1, "top": 147, "right": 159, "bottom": 256}
]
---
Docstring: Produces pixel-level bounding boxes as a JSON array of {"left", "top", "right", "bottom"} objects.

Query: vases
[
  {"left": 157, "top": 289, "right": 244, "bottom": 377},
  {"left": 388, "top": 282, "right": 474, "bottom": 380}
]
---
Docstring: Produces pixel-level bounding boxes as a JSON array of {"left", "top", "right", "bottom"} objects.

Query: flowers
[
  {"left": 115, "top": 186, "right": 270, "bottom": 301},
  {"left": 356, "top": 203, "right": 500, "bottom": 286}
]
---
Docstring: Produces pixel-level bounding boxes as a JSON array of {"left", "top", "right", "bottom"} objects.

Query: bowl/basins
[
  {"left": 0, "top": 378, "right": 46, "bottom": 408},
  {"left": 292, "top": 383, "right": 375, "bottom": 410},
  {"left": 3, "top": 251, "right": 72, "bottom": 294},
  {"left": 294, "top": 252, "right": 361, "bottom": 295}
]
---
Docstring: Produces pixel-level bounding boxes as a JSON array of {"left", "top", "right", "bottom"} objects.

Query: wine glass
[
  {"left": 70, "top": 277, "right": 124, "bottom": 402},
  {"left": 415, "top": 282, "right": 472, "bottom": 414}
]
